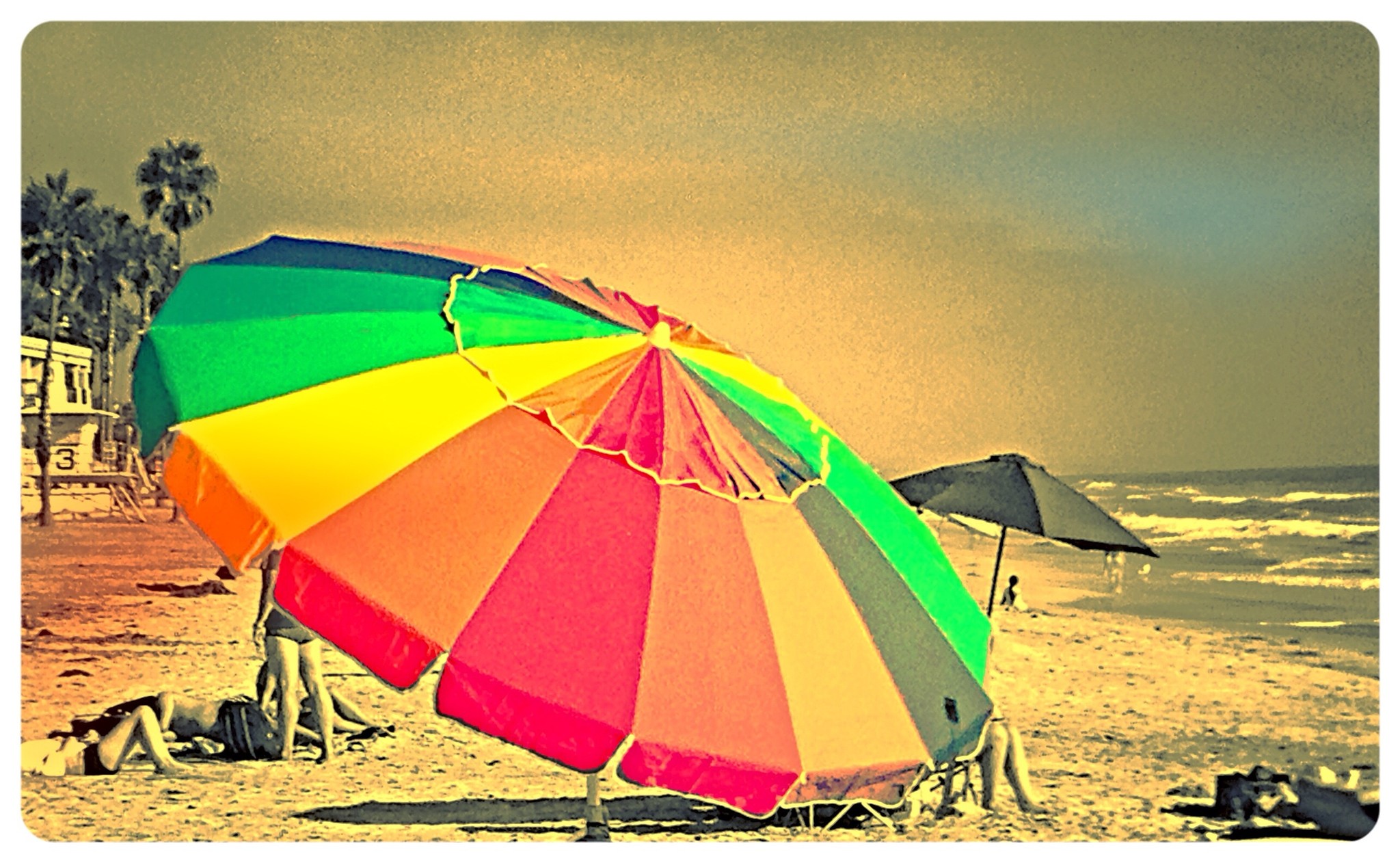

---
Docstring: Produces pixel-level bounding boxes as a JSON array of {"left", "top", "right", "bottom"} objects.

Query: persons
[
  {"left": 20, "top": 550, "right": 396, "bottom": 783},
  {"left": 977, "top": 548, "right": 1128, "bottom": 816}
]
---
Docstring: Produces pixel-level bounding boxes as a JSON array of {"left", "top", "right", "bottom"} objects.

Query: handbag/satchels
[{"left": 1215, "top": 765, "right": 1289, "bottom": 818}]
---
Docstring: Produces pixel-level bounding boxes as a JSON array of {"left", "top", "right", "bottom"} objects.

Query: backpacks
[{"left": 220, "top": 694, "right": 282, "bottom": 761}]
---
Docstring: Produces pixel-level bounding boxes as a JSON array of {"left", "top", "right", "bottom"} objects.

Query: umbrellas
[
  {"left": 882, "top": 453, "right": 1161, "bottom": 619},
  {"left": 130, "top": 230, "right": 996, "bottom": 820}
]
